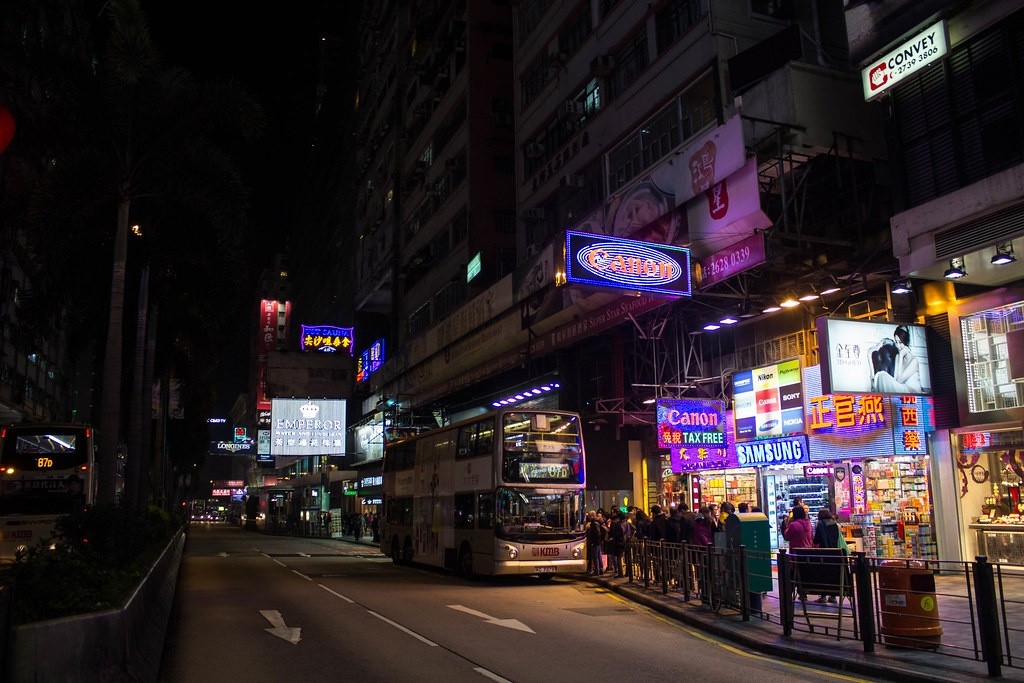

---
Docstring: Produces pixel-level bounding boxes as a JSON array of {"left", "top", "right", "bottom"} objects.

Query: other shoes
[
  {"left": 798, "top": 596, "right": 807, "bottom": 601},
  {"left": 816, "top": 598, "right": 827, "bottom": 603},
  {"left": 828, "top": 597, "right": 837, "bottom": 603}
]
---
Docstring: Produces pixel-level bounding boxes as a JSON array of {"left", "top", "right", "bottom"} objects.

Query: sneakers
[
  {"left": 600, "top": 569, "right": 604, "bottom": 575},
  {"left": 624, "top": 572, "right": 629, "bottom": 577},
  {"left": 614, "top": 571, "right": 623, "bottom": 578},
  {"left": 591, "top": 569, "right": 598, "bottom": 576}
]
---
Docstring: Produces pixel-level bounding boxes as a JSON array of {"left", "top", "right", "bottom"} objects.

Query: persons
[
  {"left": 571, "top": 502, "right": 761, "bottom": 605},
  {"left": 782, "top": 507, "right": 814, "bottom": 601},
  {"left": 789, "top": 498, "right": 809, "bottom": 521},
  {"left": 326, "top": 513, "right": 332, "bottom": 530},
  {"left": 813, "top": 509, "right": 850, "bottom": 603},
  {"left": 692, "top": 507, "right": 717, "bottom": 547},
  {"left": 363, "top": 511, "right": 382, "bottom": 542},
  {"left": 872, "top": 325, "right": 922, "bottom": 393},
  {"left": 647, "top": 505, "right": 667, "bottom": 583}
]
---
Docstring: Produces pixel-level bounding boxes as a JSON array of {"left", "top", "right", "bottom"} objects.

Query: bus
[
  {"left": 378, "top": 405, "right": 588, "bottom": 580},
  {"left": 0, "top": 422, "right": 99, "bottom": 568}
]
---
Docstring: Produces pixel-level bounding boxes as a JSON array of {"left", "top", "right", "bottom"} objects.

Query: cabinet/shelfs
[
  {"left": 698, "top": 461, "right": 929, "bottom": 536},
  {"left": 964, "top": 303, "right": 1024, "bottom": 413}
]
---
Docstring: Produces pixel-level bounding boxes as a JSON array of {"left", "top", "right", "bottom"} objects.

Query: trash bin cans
[
  {"left": 723, "top": 511, "right": 774, "bottom": 594},
  {"left": 877, "top": 558, "right": 944, "bottom": 648}
]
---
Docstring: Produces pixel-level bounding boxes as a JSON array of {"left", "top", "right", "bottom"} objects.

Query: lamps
[
  {"left": 891, "top": 277, "right": 912, "bottom": 294},
  {"left": 990, "top": 240, "right": 1017, "bottom": 265},
  {"left": 943, "top": 256, "right": 968, "bottom": 279}
]
